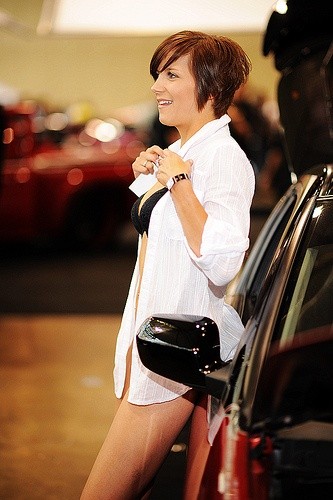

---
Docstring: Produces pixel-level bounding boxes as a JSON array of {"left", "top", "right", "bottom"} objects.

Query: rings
[{"left": 144, "top": 160, "right": 148, "bottom": 166}]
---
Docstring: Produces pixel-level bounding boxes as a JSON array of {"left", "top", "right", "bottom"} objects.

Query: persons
[
  {"left": 79, "top": 29, "right": 256, "bottom": 500},
  {"left": 276, "top": 44, "right": 331, "bottom": 186}
]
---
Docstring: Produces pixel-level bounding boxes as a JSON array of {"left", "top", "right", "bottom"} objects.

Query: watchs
[{"left": 166, "top": 173, "right": 191, "bottom": 193}]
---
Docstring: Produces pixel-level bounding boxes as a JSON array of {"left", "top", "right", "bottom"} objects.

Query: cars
[{"left": 137, "top": 163, "right": 333, "bottom": 500}]
